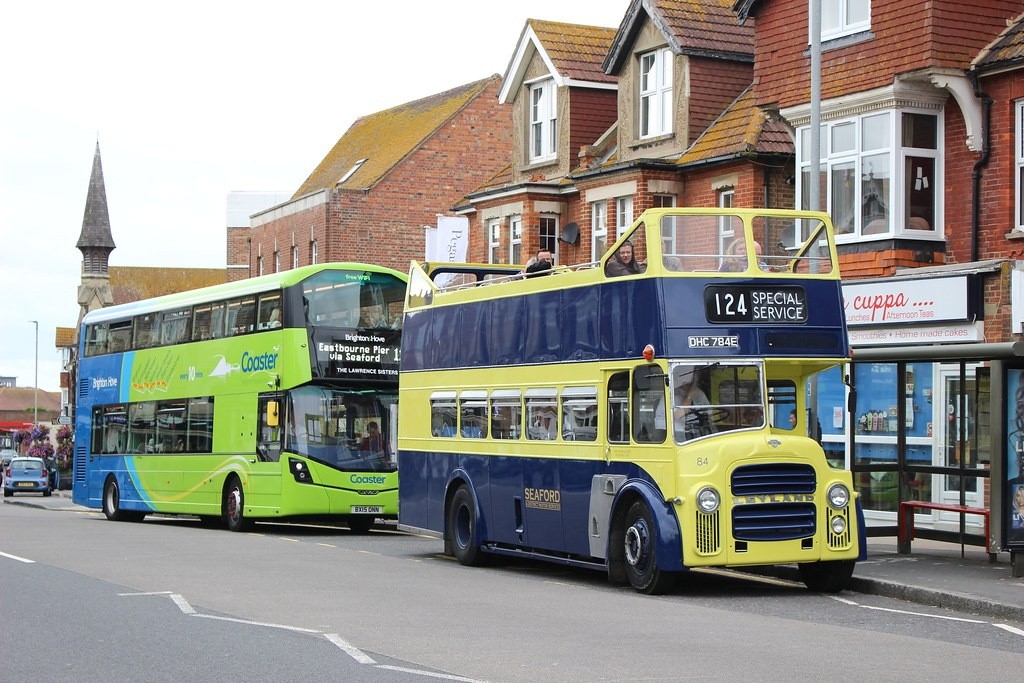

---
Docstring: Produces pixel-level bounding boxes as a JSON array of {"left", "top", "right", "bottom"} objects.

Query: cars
[
  {"left": 3, "top": 457, "right": 60, "bottom": 497},
  {"left": 0, "top": 448, "right": 19, "bottom": 467}
]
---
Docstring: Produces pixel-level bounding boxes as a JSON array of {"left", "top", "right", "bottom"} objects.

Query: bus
[
  {"left": 71, "top": 261, "right": 408, "bottom": 537},
  {"left": 393, "top": 205, "right": 869, "bottom": 597}
]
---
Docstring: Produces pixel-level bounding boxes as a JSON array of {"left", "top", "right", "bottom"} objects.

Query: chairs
[{"left": 553, "top": 265, "right": 573, "bottom": 274}]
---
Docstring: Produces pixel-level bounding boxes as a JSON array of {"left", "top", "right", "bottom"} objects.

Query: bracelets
[{"left": 685, "top": 396, "right": 692, "bottom": 401}]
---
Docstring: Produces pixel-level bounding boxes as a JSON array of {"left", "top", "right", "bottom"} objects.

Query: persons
[
  {"left": 268, "top": 309, "right": 283, "bottom": 329},
  {"left": 1012, "top": 484, "right": 1024, "bottom": 522},
  {"left": 606, "top": 240, "right": 643, "bottom": 277},
  {"left": 719, "top": 238, "right": 768, "bottom": 271},
  {"left": 360, "top": 421, "right": 387, "bottom": 452},
  {"left": 522, "top": 248, "right": 574, "bottom": 280},
  {"left": 654, "top": 373, "right": 714, "bottom": 442},
  {"left": 641, "top": 237, "right": 666, "bottom": 273},
  {"left": 356, "top": 307, "right": 372, "bottom": 328},
  {"left": 788, "top": 409, "right": 821, "bottom": 447}
]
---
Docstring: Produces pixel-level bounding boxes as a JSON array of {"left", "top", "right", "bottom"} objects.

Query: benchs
[{"left": 900, "top": 500, "right": 990, "bottom": 553}]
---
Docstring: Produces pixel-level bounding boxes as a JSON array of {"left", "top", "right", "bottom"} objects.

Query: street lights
[{"left": 27, "top": 319, "right": 38, "bottom": 428}]
